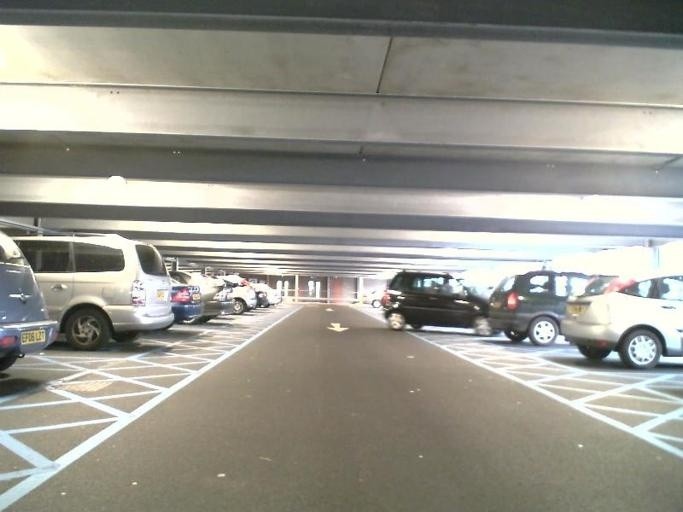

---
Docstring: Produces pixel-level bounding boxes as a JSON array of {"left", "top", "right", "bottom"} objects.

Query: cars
[
  {"left": 164, "top": 269, "right": 285, "bottom": 325},
  {"left": 362, "top": 286, "right": 384, "bottom": 308}
]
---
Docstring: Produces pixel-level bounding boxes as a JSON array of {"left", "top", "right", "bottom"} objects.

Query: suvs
[
  {"left": 0, "top": 228, "right": 60, "bottom": 370},
  {"left": 380, "top": 268, "right": 492, "bottom": 338},
  {"left": 560, "top": 266, "right": 683, "bottom": 369},
  {"left": 486, "top": 266, "right": 610, "bottom": 345}
]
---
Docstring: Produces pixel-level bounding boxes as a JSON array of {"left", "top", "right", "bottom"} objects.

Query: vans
[{"left": 9, "top": 233, "right": 175, "bottom": 352}]
